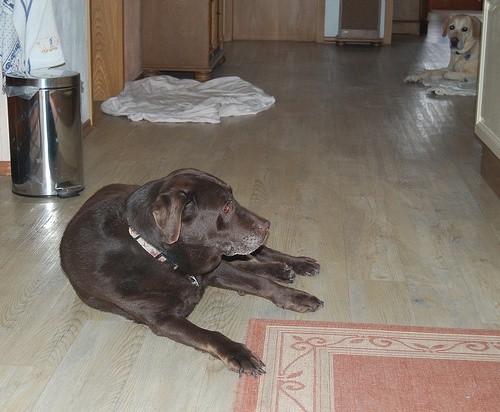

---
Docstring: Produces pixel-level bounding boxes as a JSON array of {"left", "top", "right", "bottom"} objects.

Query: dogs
[
  {"left": 59, "top": 167, "right": 326, "bottom": 378},
  {"left": 403, "top": 14, "right": 483, "bottom": 84}
]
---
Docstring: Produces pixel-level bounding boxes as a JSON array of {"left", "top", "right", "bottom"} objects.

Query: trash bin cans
[{"left": 7, "top": 69, "right": 85, "bottom": 199}]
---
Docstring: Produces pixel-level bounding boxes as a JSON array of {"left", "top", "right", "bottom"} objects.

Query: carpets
[{"left": 234, "top": 320, "right": 500, "bottom": 412}]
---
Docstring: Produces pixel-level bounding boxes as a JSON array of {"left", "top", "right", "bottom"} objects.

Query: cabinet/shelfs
[{"left": 141, "top": 0, "right": 227, "bottom": 81}]
